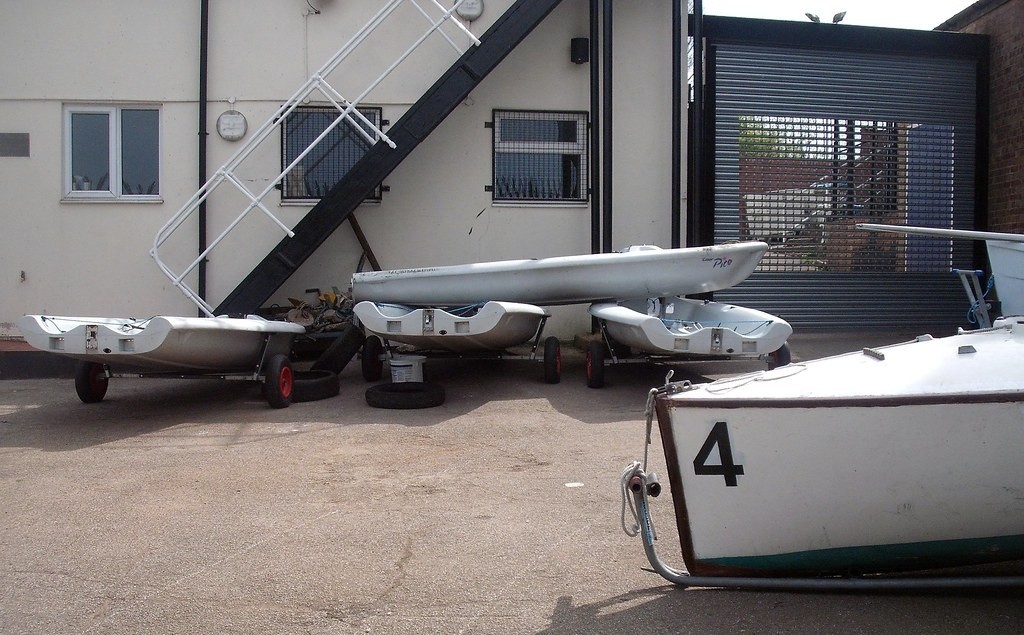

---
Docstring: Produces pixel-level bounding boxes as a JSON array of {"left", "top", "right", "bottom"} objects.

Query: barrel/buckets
[{"left": 390, "top": 358, "right": 424, "bottom": 383}]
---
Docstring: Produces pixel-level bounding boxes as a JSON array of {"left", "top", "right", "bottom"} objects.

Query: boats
[
  {"left": 352, "top": 300, "right": 544, "bottom": 351},
  {"left": 587, "top": 296, "right": 793, "bottom": 356},
  {"left": 652, "top": 314, "right": 1024, "bottom": 581},
  {"left": 17, "top": 314, "right": 306, "bottom": 376},
  {"left": 351, "top": 241, "right": 770, "bottom": 309}
]
